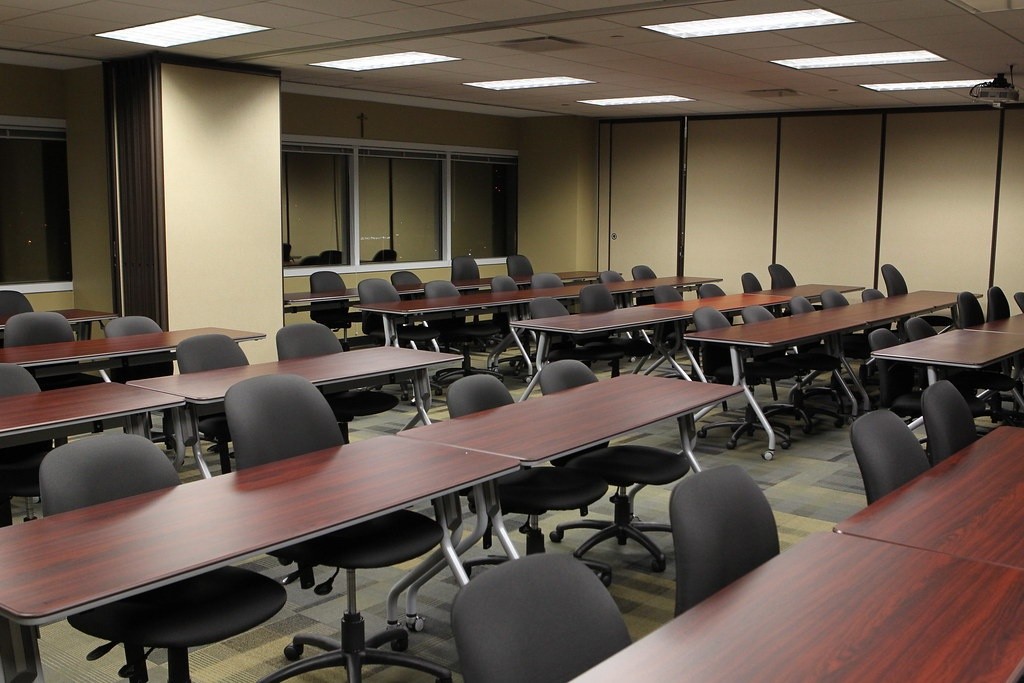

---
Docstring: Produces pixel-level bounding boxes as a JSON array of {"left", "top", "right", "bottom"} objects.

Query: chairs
[{"left": 0, "top": 252, "right": 1024, "bottom": 683}]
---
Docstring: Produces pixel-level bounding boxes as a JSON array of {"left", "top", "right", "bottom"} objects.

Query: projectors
[{"left": 978, "top": 87, "right": 1020, "bottom": 102}]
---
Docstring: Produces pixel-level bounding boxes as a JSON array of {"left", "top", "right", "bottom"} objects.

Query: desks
[
  {"left": 353, "top": 275, "right": 724, "bottom": 404},
  {"left": 0, "top": 309, "right": 118, "bottom": 341},
  {"left": 396, "top": 374, "right": 746, "bottom": 632},
  {"left": 567, "top": 534, "right": 1024, "bottom": 683},
  {"left": 0, "top": 432, "right": 520, "bottom": 683},
  {"left": 0, "top": 327, "right": 267, "bottom": 383},
  {"left": 0, "top": 345, "right": 464, "bottom": 481},
  {"left": 510, "top": 284, "right": 866, "bottom": 403},
  {"left": 870, "top": 313, "right": 1024, "bottom": 432},
  {"left": 283, "top": 270, "right": 623, "bottom": 313},
  {"left": 683, "top": 291, "right": 984, "bottom": 462},
  {"left": 832, "top": 425, "right": 1024, "bottom": 569}
]
